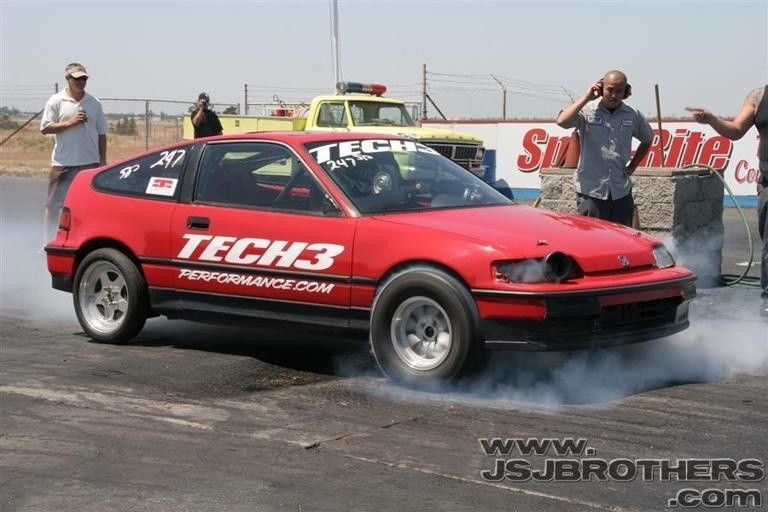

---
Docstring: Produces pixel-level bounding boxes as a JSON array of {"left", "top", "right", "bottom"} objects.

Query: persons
[
  {"left": 190, "top": 93, "right": 224, "bottom": 138},
  {"left": 322, "top": 160, "right": 430, "bottom": 213},
  {"left": 39, "top": 62, "right": 107, "bottom": 242},
  {"left": 556, "top": 70, "right": 654, "bottom": 227},
  {"left": 685, "top": 85, "right": 768, "bottom": 317}
]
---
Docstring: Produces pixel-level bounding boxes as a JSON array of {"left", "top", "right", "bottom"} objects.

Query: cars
[{"left": 44, "top": 130, "right": 698, "bottom": 393}]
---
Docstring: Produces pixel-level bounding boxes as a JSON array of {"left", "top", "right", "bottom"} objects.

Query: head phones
[{"left": 597, "top": 77, "right": 632, "bottom": 100}]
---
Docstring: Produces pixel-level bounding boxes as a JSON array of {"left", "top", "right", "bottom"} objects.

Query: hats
[{"left": 65, "top": 61, "right": 90, "bottom": 79}]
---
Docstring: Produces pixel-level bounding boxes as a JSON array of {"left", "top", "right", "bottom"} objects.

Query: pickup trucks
[{"left": 182, "top": 82, "right": 486, "bottom": 179}]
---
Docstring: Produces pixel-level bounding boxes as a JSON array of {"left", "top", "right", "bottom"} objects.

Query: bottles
[{"left": 78, "top": 107, "right": 86, "bottom": 114}]
